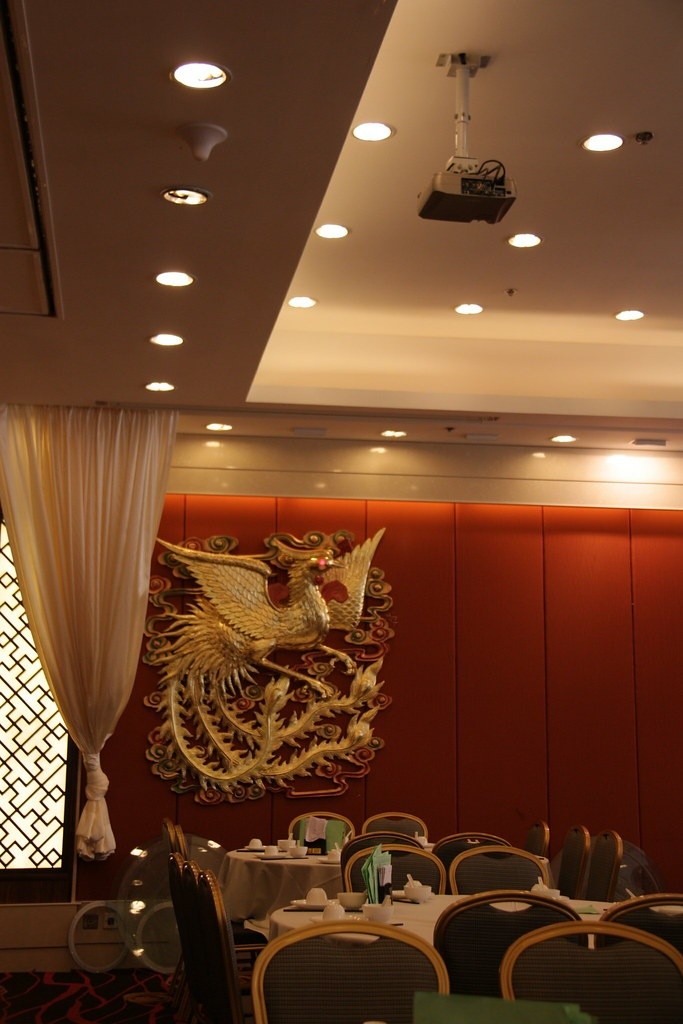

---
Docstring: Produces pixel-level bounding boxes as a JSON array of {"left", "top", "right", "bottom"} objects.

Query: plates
[
  {"left": 254, "top": 853, "right": 286, "bottom": 859},
  {"left": 309, "top": 915, "right": 358, "bottom": 924},
  {"left": 245, "top": 845, "right": 266, "bottom": 851},
  {"left": 316, "top": 857, "right": 340, "bottom": 864},
  {"left": 290, "top": 900, "right": 336, "bottom": 911}
]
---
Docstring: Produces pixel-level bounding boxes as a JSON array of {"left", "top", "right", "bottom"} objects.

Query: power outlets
[{"left": 103, "top": 913, "right": 119, "bottom": 929}]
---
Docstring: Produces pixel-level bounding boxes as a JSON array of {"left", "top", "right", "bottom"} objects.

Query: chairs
[{"left": 166, "top": 812, "right": 683, "bottom": 1024}]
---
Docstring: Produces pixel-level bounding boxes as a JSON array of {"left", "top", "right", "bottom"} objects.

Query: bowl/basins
[
  {"left": 278, "top": 840, "right": 296, "bottom": 852},
  {"left": 337, "top": 892, "right": 367, "bottom": 910},
  {"left": 362, "top": 902, "right": 393, "bottom": 923},
  {"left": 288, "top": 846, "right": 308, "bottom": 857},
  {"left": 530, "top": 888, "right": 559, "bottom": 898},
  {"left": 403, "top": 886, "right": 432, "bottom": 903}
]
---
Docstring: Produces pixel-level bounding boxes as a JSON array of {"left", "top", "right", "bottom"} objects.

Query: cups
[
  {"left": 249, "top": 838, "right": 262, "bottom": 847},
  {"left": 305, "top": 888, "right": 327, "bottom": 904},
  {"left": 419, "top": 836, "right": 427, "bottom": 846},
  {"left": 328, "top": 849, "right": 340, "bottom": 861},
  {"left": 264, "top": 846, "right": 278, "bottom": 855},
  {"left": 323, "top": 902, "right": 346, "bottom": 922}
]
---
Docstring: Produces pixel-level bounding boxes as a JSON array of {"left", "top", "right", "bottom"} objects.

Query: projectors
[{"left": 418, "top": 172, "right": 517, "bottom": 224}]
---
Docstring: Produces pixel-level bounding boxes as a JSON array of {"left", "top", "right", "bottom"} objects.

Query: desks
[
  {"left": 269, "top": 894, "right": 683, "bottom": 949},
  {"left": 218, "top": 844, "right": 557, "bottom": 939}
]
